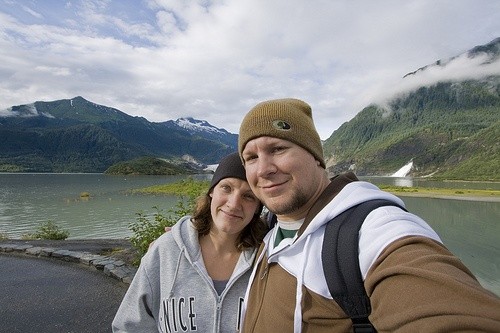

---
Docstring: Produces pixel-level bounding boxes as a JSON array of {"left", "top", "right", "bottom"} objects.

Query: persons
[
  {"left": 141, "top": 98, "right": 500, "bottom": 333},
  {"left": 111, "top": 153, "right": 267, "bottom": 333}
]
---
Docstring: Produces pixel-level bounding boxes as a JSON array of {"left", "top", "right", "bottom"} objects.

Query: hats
[
  {"left": 238, "top": 97, "right": 325, "bottom": 170},
  {"left": 207, "top": 152, "right": 248, "bottom": 196}
]
